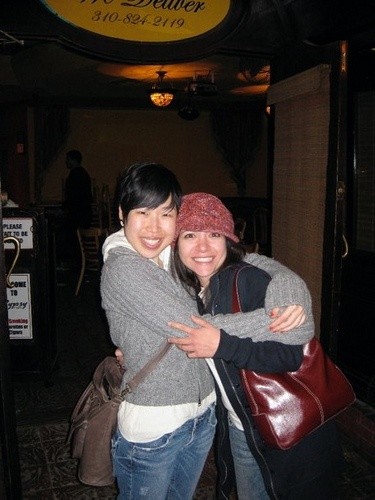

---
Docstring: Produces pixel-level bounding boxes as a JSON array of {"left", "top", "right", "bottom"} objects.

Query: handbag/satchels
[
  {"left": 232, "top": 264, "right": 357, "bottom": 451},
  {"left": 64, "top": 355, "right": 127, "bottom": 488}
]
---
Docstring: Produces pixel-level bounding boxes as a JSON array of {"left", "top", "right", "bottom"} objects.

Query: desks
[{"left": 37, "top": 201, "right": 73, "bottom": 287}]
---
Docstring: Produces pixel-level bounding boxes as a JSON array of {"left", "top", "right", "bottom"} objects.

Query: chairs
[
  {"left": 74, "top": 194, "right": 121, "bottom": 295},
  {"left": 252, "top": 207, "right": 268, "bottom": 255}
]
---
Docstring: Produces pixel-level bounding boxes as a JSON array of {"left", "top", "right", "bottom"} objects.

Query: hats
[{"left": 170, "top": 192, "right": 240, "bottom": 247}]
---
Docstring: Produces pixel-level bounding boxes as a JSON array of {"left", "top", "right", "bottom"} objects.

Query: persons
[
  {"left": 59, "top": 149, "right": 94, "bottom": 286},
  {"left": 100, "top": 165, "right": 315, "bottom": 500},
  {"left": 115, "top": 193, "right": 344, "bottom": 500}
]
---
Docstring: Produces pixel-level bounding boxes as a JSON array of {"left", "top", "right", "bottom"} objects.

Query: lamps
[{"left": 145, "top": 70, "right": 178, "bottom": 109}]
